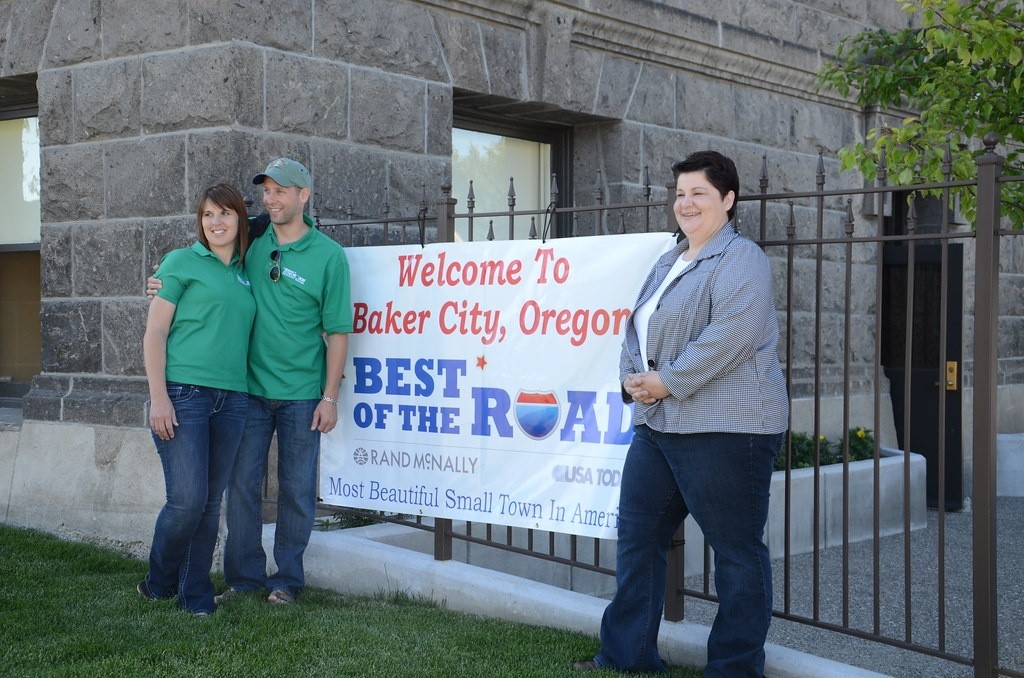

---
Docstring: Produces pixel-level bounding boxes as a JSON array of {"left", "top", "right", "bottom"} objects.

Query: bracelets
[{"left": 320, "top": 394, "right": 339, "bottom": 406}]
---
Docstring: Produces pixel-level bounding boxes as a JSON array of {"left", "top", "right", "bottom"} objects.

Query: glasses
[{"left": 269, "top": 248, "right": 281, "bottom": 282}]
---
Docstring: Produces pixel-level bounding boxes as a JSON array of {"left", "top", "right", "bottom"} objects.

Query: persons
[
  {"left": 146, "top": 156, "right": 352, "bottom": 606},
  {"left": 571, "top": 151, "right": 793, "bottom": 677},
  {"left": 135, "top": 182, "right": 257, "bottom": 620}
]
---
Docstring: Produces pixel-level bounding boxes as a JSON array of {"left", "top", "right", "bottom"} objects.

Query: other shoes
[{"left": 268, "top": 590, "right": 296, "bottom": 606}]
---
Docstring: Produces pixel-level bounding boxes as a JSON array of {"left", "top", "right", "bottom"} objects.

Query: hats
[{"left": 251, "top": 157, "right": 312, "bottom": 189}]
